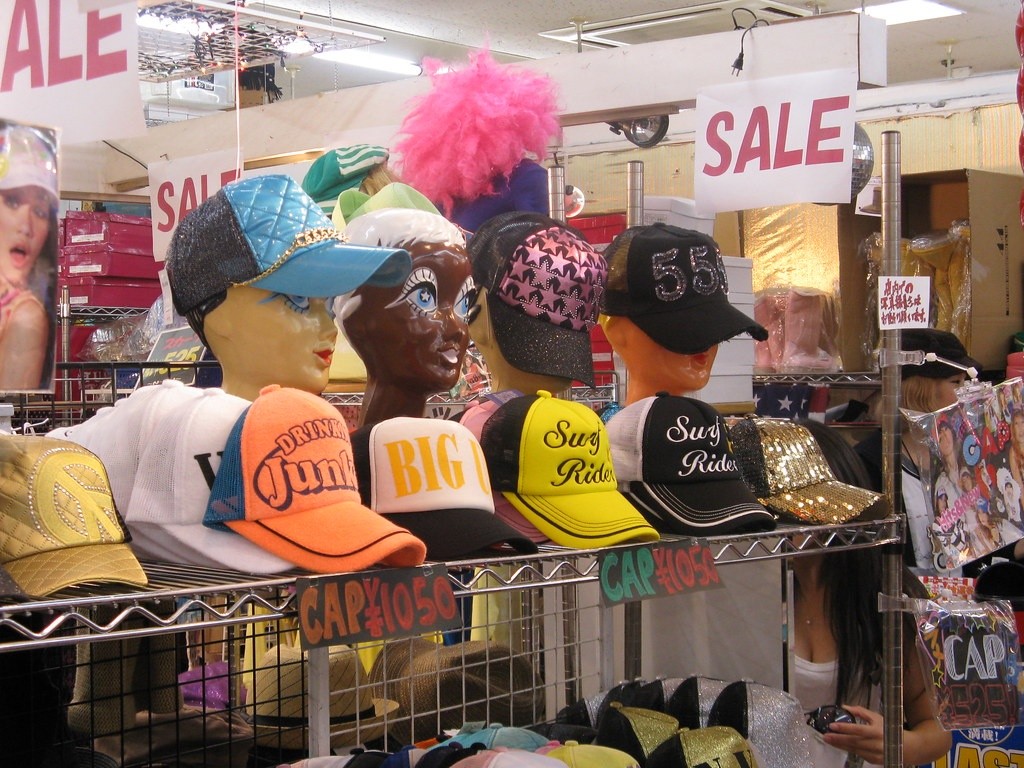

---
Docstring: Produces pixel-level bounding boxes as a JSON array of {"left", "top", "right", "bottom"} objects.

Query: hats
[
  {"left": 0, "top": 435, "right": 149, "bottom": 597},
  {"left": 276, "top": 673, "right": 813, "bottom": 768},
  {"left": 301, "top": 144, "right": 389, "bottom": 217},
  {"left": 46, "top": 379, "right": 296, "bottom": 573},
  {"left": 481, "top": 390, "right": 660, "bottom": 550},
  {"left": 349, "top": 416, "right": 538, "bottom": 562},
  {"left": 459, "top": 398, "right": 550, "bottom": 545},
  {"left": 731, "top": 414, "right": 892, "bottom": 526},
  {"left": 165, "top": 174, "right": 413, "bottom": 316},
  {"left": 369, "top": 639, "right": 544, "bottom": 749},
  {"left": 603, "top": 391, "right": 776, "bottom": 537},
  {"left": 600, "top": 221, "right": 769, "bottom": 354},
  {"left": 878, "top": 328, "right": 982, "bottom": 382},
  {"left": 0, "top": 126, "right": 60, "bottom": 210},
  {"left": 204, "top": 385, "right": 428, "bottom": 574},
  {"left": 240, "top": 644, "right": 399, "bottom": 749},
  {"left": 468, "top": 211, "right": 609, "bottom": 388},
  {"left": 332, "top": 183, "right": 443, "bottom": 233}
]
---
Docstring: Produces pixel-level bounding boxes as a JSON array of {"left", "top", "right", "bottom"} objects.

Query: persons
[
  {"left": 787, "top": 418, "right": 954, "bottom": 768},
  {"left": 167, "top": 175, "right": 411, "bottom": 403},
  {"left": 335, "top": 207, "right": 476, "bottom": 426},
  {"left": 853, "top": 327, "right": 1023, "bottom": 577},
  {"left": 602, "top": 224, "right": 770, "bottom": 407},
  {"left": 0, "top": 127, "right": 56, "bottom": 390},
  {"left": 465, "top": 211, "right": 608, "bottom": 399}
]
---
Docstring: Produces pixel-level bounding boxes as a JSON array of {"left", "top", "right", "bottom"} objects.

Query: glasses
[{"left": 807, "top": 706, "right": 856, "bottom": 734}]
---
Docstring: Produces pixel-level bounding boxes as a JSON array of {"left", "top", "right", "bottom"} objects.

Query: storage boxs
[
  {"left": 48, "top": 211, "right": 165, "bottom": 427},
  {"left": 899, "top": 167, "right": 1024, "bottom": 374}
]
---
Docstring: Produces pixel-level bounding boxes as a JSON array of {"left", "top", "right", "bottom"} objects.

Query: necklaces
[
  {"left": 902, "top": 441, "right": 931, "bottom": 490},
  {"left": 803, "top": 615, "right": 815, "bottom": 625}
]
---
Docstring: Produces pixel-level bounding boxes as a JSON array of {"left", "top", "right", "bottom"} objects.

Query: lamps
[{"left": 603, "top": 112, "right": 670, "bottom": 149}]
